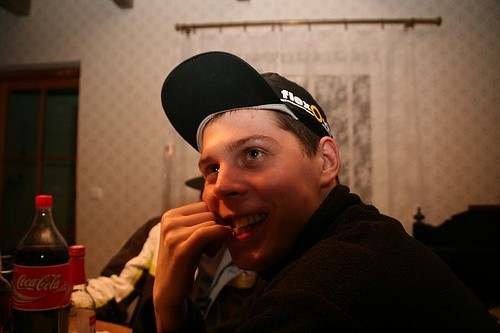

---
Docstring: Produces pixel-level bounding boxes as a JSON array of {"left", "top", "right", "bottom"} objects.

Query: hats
[
  {"left": 161, "top": 51, "right": 334, "bottom": 154},
  {"left": 184, "top": 176, "right": 205, "bottom": 191}
]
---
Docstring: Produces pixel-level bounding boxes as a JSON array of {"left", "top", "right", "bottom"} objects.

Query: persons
[
  {"left": 72, "top": 177, "right": 258, "bottom": 330},
  {"left": 154, "top": 51, "right": 500, "bottom": 333}
]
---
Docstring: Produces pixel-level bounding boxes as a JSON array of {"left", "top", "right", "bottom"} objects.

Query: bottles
[
  {"left": 68, "top": 245, "right": 97, "bottom": 333},
  {"left": 12, "top": 195, "right": 69, "bottom": 333}
]
